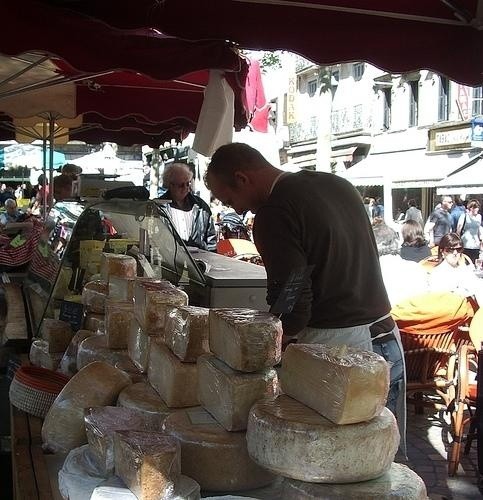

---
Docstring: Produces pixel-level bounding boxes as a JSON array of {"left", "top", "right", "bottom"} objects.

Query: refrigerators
[{"left": 153, "top": 241, "right": 271, "bottom": 310}]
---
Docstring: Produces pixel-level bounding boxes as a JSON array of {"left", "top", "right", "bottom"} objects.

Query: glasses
[{"left": 444, "top": 247, "right": 464, "bottom": 254}]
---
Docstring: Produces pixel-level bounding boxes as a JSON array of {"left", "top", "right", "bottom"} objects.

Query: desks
[
  {"left": 9, "top": 353, "right": 66, "bottom": 500},
  {"left": 0, "top": 279, "right": 28, "bottom": 347}
]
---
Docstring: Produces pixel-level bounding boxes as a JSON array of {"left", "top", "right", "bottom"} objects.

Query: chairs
[
  {"left": 420, "top": 253, "right": 474, "bottom": 270},
  {"left": 447, "top": 344, "right": 477, "bottom": 477},
  {"left": 394, "top": 294, "right": 471, "bottom": 415}
]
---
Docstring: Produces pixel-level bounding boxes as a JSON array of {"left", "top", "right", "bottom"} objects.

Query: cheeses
[{"left": 29, "top": 252, "right": 430, "bottom": 499}]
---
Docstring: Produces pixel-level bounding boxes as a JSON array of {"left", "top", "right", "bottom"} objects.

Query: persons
[
  {"left": 203, "top": 142, "right": 413, "bottom": 470},
  {"left": 3, "top": 162, "right": 483, "bottom": 273}
]
